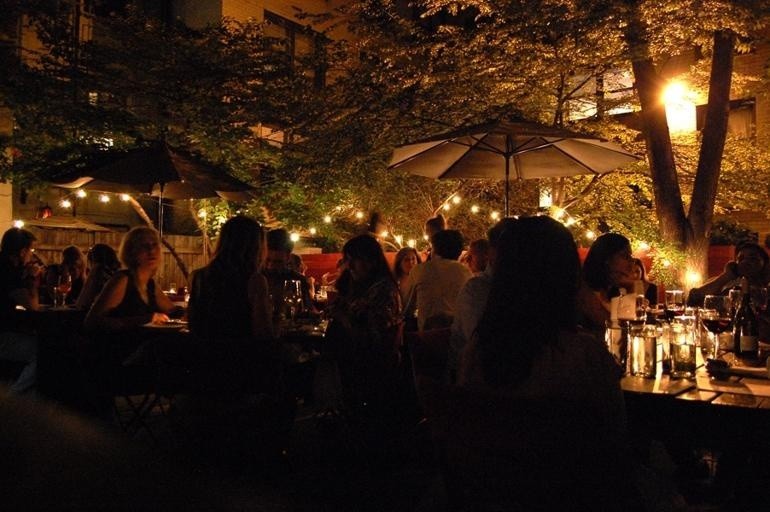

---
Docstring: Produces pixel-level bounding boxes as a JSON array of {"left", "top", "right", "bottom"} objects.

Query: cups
[
  {"left": 170, "top": 283, "right": 177, "bottom": 292},
  {"left": 664, "top": 290, "right": 686, "bottom": 318},
  {"left": 603, "top": 315, "right": 696, "bottom": 381}
]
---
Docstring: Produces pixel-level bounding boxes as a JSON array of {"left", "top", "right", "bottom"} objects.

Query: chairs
[{"left": 111, "top": 390, "right": 168, "bottom": 440}]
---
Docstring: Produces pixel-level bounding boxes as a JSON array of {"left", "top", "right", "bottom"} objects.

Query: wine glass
[
  {"left": 282, "top": 280, "right": 303, "bottom": 315},
  {"left": 698, "top": 291, "right": 734, "bottom": 366},
  {"left": 45, "top": 272, "right": 72, "bottom": 309}
]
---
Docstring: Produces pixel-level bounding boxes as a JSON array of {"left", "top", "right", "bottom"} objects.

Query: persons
[
  {"left": 1, "top": 214, "right": 658, "bottom": 511},
  {"left": 687, "top": 244, "right": 770, "bottom": 305},
  {"left": 359, "top": 211, "right": 381, "bottom": 240}
]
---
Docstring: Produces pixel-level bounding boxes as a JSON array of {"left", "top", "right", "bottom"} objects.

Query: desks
[
  {"left": 621, "top": 355, "right": 769, "bottom": 414},
  {"left": 15, "top": 301, "right": 80, "bottom": 315}
]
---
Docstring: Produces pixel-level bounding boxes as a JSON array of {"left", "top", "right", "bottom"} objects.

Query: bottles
[{"left": 737, "top": 294, "right": 759, "bottom": 359}]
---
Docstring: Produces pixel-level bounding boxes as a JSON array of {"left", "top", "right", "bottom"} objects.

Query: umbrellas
[
  {"left": 49, "top": 139, "right": 257, "bottom": 246},
  {"left": 386, "top": 117, "right": 644, "bottom": 221}
]
